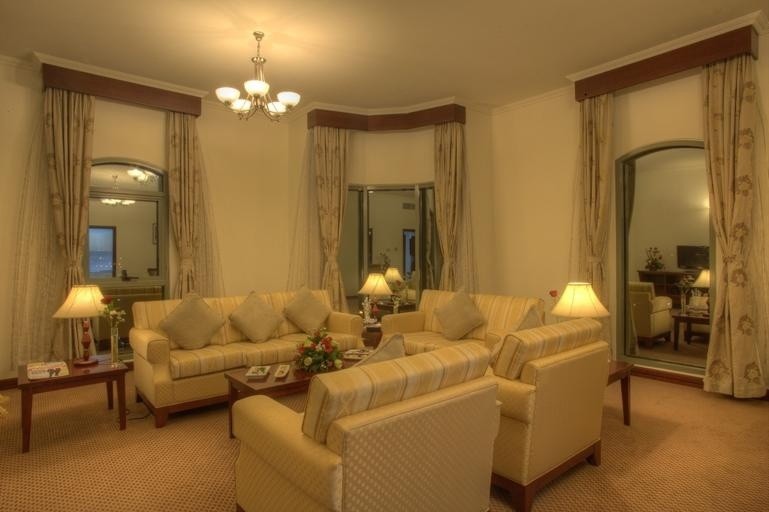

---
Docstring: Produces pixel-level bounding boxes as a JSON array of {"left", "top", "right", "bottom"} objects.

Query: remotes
[{"left": 274, "top": 365, "right": 290, "bottom": 378}]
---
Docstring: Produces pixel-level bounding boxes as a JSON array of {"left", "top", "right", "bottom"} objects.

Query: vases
[{"left": 110, "top": 327, "right": 119, "bottom": 367}]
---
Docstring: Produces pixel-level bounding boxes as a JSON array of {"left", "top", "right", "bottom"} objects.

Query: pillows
[
  {"left": 431, "top": 285, "right": 486, "bottom": 341},
  {"left": 281, "top": 285, "right": 331, "bottom": 335},
  {"left": 157, "top": 290, "right": 226, "bottom": 349},
  {"left": 351, "top": 332, "right": 407, "bottom": 371},
  {"left": 228, "top": 290, "right": 284, "bottom": 343}
]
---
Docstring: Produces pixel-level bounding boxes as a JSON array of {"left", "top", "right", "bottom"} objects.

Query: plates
[{"left": 274, "top": 364, "right": 290, "bottom": 379}]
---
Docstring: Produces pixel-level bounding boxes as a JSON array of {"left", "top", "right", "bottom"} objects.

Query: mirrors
[
  {"left": 366, "top": 185, "right": 421, "bottom": 301},
  {"left": 337, "top": 184, "right": 364, "bottom": 297},
  {"left": 613, "top": 138, "right": 725, "bottom": 372},
  {"left": 421, "top": 184, "right": 445, "bottom": 291},
  {"left": 87, "top": 159, "right": 169, "bottom": 285}
]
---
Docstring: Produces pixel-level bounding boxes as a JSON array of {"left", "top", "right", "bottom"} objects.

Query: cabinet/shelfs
[{"left": 638, "top": 269, "right": 707, "bottom": 309}]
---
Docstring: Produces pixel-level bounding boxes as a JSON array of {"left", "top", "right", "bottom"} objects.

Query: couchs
[
  {"left": 374, "top": 289, "right": 546, "bottom": 375},
  {"left": 628, "top": 280, "right": 673, "bottom": 347},
  {"left": 489, "top": 318, "right": 610, "bottom": 512},
  {"left": 127, "top": 289, "right": 366, "bottom": 428},
  {"left": 228, "top": 341, "right": 499, "bottom": 512}
]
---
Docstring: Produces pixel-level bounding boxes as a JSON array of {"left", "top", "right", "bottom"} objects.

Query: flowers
[
  {"left": 645, "top": 245, "right": 665, "bottom": 271},
  {"left": 290, "top": 326, "right": 346, "bottom": 373},
  {"left": 98, "top": 293, "right": 128, "bottom": 361},
  {"left": 549, "top": 289, "right": 565, "bottom": 324}
]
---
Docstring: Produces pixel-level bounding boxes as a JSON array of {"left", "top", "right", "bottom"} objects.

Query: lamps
[
  {"left": 548, "top": 282, "right": 612, "bottom": 319},
  {"left": 52, "top": 284, "right": 112, "bottom": 367},
  {"left": 356, "top": 273, "right": 395, "bottom": 331},
  {"left": 100, "top": 174, "right": 136, "bottom": 209},
  {"left": 385, "top": 267, "right": 406, "bottom": 287},
  {"left": 127, "top": 165, "right": 161, "bottom": 185},
  {"left": 214, "top": 30, "right": 303, "bottom": 123},
  {"left": 691, "top": 269, "right": 711, "bottom": 298}
]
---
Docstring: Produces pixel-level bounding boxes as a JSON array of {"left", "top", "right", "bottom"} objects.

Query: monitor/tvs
[{"left": 677, "top": 245, "right": 709, "bottom": 269}]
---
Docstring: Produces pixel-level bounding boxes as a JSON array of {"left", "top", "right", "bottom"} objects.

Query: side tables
[
  {"left": 16, "top": 355, "right": 129, "bottom": 454},
  {"left": 604, "top": 357, "right": 635, "bottom": 428},
  {"left": 672, "top": 308, "right": 711, "bottom": 351}
]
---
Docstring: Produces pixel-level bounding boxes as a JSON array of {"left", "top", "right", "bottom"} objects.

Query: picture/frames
[{"left": 151, "top": 223, "right": 158, "bottom": 244}]
